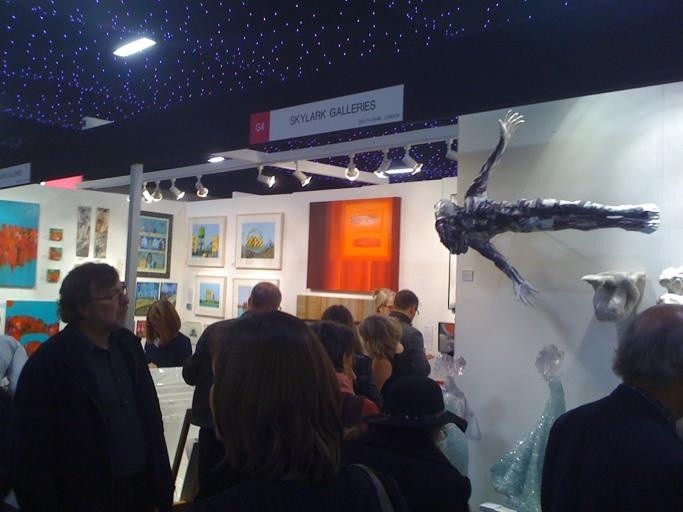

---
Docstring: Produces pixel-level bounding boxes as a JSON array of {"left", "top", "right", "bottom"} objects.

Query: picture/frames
[
  {"left": 448, "top": 193, "right": 457, "bottom": 311},
  {"left": 437, "top": 322, "right": 455, "bottom": 358},
  {"left": 182, "top": 321, "right": 202, "bottom": 344},
  {"left": 234, "top": 212, "right": 284, "bottom": 271},
  {"left": 135, "top": 211, "right": 174, "bottom": 279},
  {"left": 233, "top": 277, "right": 281, "bottom": 319},
  {"left": 187, "top": 215, "right": 227, "bottom": 268},
  {"left": 193, "top": 274, "right": 227, "bottom": 318},
  {"left": 134, "top": 282, "right": 178, "bottom": 317},
  {"left": 136, "top": 320, "right": 150, "bottom": 339}
]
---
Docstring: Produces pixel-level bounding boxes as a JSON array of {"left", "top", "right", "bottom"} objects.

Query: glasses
[
  {"left": 91, "top": 281, "right": 129, "bottom": 302},
  {"left": 383, "top": 304, "right": 394, "bottom": 310},
  {"left": 415, "top": 309, "right": 420, "bottom": 315}
]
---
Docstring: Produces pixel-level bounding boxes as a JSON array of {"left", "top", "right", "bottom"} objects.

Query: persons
[
  {"left": 2, "top": 334, "right": 26, "bottom": 509},
  {"left": 297, "top": 322, "right": 374, "bottom": 442},
  {"left": 138, "top": 299, "right": 192, "bottom": 369},
  {"left": 309, "top": 376, "right": 471, "bottom": 512},
  {"left": 183, "top": 281, "right": 284, "bottom": 488},
  {"left": 541, "top": 303, "right": 682, "bottom": 512},
  {"left": 318, "top": 306, "right": 379, "bottom": 407},
  {"left": 372, "top": 290, "right": 430, "bottom": 374},
  {"left": 181, "top": 310, "right": 342, "bottom": 510},
  {"left": 359, "top": 316, "right": 407, "bottom": 386},
  {"left": 433, "top": 111, "right": 660, "bottom": 305},
  {"left": 10, "top": 262, "right": 175, "bottom": 510}
]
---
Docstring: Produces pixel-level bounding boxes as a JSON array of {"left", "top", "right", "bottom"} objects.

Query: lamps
[
  {"left": 373, "top": 149, "right": 392, "bottom": 179},
  {"left": 151, "top": 180, "right": 163, "bottom": 203},
  {"left": 402, "top": 145, "right": 424, "bottom": 174},
  {"left": 293, "top": 160, "right": 312, "bottom": 187},
  {"left": 345, "top": 154, "right": 360, "bottom": 180},
  {"left": 142, "top": 180, "right": 152, "bottom": 204},
  {"left": 169, "top": 179, "right": 185, "bottom": 201},
  {"left": 195, "top": 176, "right": 209, "bottom": 198},
  {"left": 257, "top": 166, "right": 276, "bottom": 189},
  {"left": 446, "top": 140, "right": 458, "bottom": 161}
]
